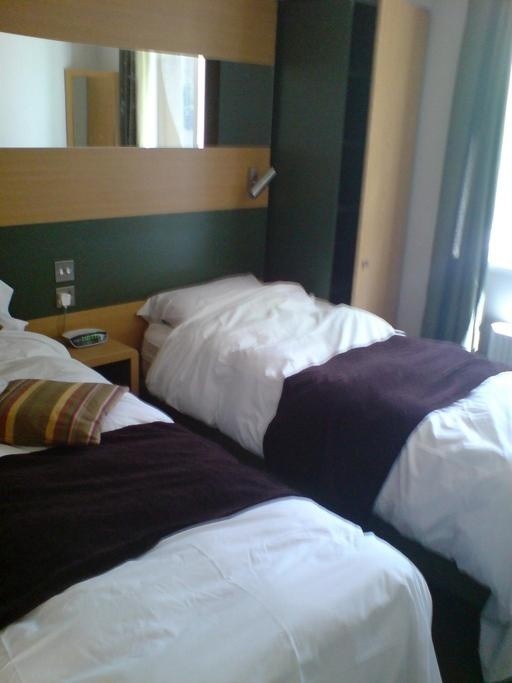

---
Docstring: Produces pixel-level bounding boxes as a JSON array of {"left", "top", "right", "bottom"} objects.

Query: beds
[
  {"left": 0, "top": 324, "right": 434, "bottom": 678},
  {"left": 145, "top": 280, "right": 510, "bottom": 576}
]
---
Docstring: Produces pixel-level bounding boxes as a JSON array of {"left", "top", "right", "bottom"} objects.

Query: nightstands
[{"left": 53, "top": 336, "right": 139, "bottom": 397}]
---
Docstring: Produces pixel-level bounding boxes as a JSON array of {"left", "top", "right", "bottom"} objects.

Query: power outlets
[{"left": 52, "top": 287, "right": 77, "bottom": 309}]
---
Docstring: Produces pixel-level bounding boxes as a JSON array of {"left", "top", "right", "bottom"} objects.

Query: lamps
[{"left": 245, "top": 166, "right": 281, "bottom": 195}]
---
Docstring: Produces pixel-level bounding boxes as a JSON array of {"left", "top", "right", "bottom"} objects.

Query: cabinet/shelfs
[{"left": 269, "top": 4, "right": 432, "bottom": 329}]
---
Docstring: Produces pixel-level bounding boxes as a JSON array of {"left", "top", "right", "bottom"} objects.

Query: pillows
[
  {"left": 137, "top": 274, "right": 262, "bottom": 332},
  {"left": 1, "top": 378, "right": 130, "bottom": 448}
]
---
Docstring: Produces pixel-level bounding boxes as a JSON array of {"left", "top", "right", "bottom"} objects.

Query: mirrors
[{"left": 1, "top": 30, "right": 275, "bottom": 148}]
